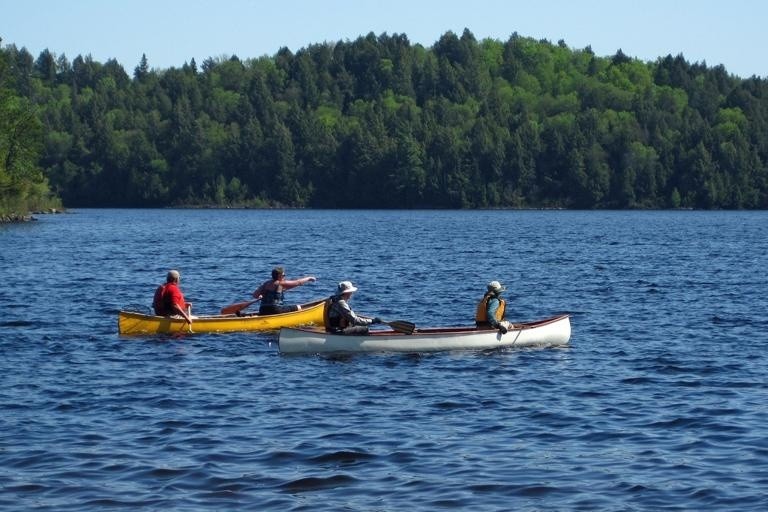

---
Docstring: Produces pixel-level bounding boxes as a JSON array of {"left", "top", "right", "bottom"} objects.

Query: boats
[
  {"left": 278, "top": 315, "right": 571, "bottom": 352},
  {"left": 119, "top": 298, "right": 341, "bottom": 335}
]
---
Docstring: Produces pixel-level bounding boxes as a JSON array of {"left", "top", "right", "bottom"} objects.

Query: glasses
[{"left": 279, "top": 274, "right": 286, "bottom": 278}]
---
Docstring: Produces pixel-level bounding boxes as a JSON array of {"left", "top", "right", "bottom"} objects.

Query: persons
[
  {"left": 323, "top": 281, "right": 380, "bottom": 335},
  {"left": 152, "top": 270, "right": 192, "bottom": 326},
  {"left": 253, "top": 268, "right": 316, "bottom": 316},
  {"left": 476, "top": 281, "right": 515, "bottom": 334}
]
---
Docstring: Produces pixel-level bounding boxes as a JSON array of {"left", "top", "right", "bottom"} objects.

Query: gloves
[
  {"left": 498, "top": 323, "right": 507, "bottom": 334},
  {"left": 373, "top": 318, "right": 382, "bottom": 324}
]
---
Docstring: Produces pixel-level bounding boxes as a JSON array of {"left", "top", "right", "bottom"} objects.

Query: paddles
[
  {"left": 186, "top": 306, "right": 194, "bottom": 333},
  {"left": 499, "top": 326, "right": 508, "bottom": 334},
  {"left": 222, "top": 288, "right": 288, "bottom": 314},
  {"left": 357, "top": 315, "right": 415, "bottom": 335}
]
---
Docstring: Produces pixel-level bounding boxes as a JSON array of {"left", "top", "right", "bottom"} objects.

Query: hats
[
  {"left": 335, "top": 281, "right": 358, "bottom": 295},
  {"left": 487, "top": 281, "right": 507, "bottom": 293}
]
